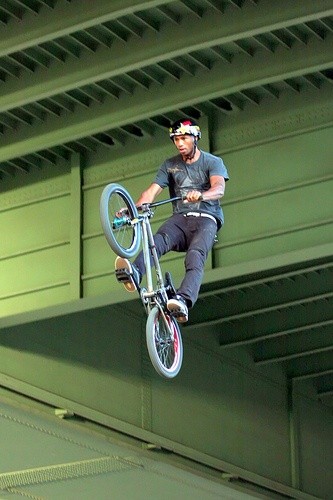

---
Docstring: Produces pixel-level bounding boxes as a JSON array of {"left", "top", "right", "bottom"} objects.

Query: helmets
[{"left": 168, "top": 118, "right": 201, "bottom": 143}]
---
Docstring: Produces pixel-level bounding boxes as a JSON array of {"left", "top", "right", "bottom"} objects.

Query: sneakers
[
  {"left": 115, "top": 256, "right": 141, "bottom": 293},
  {"left": 167, "top": 297, "right": 189, "bottom": 323}
]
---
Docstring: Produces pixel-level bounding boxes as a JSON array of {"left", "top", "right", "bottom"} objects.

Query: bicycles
[{"left": 99, "top": 181, "right": 204, "bottom": 379}]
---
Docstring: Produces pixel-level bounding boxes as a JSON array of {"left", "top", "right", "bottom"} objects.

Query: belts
[{"left": 183, "top": 211, "right": 218, "bottom": 224}]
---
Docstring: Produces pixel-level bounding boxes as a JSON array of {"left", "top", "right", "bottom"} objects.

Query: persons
[{"left": 114, "top": 117, "right": 230, "bottom": 323}]
